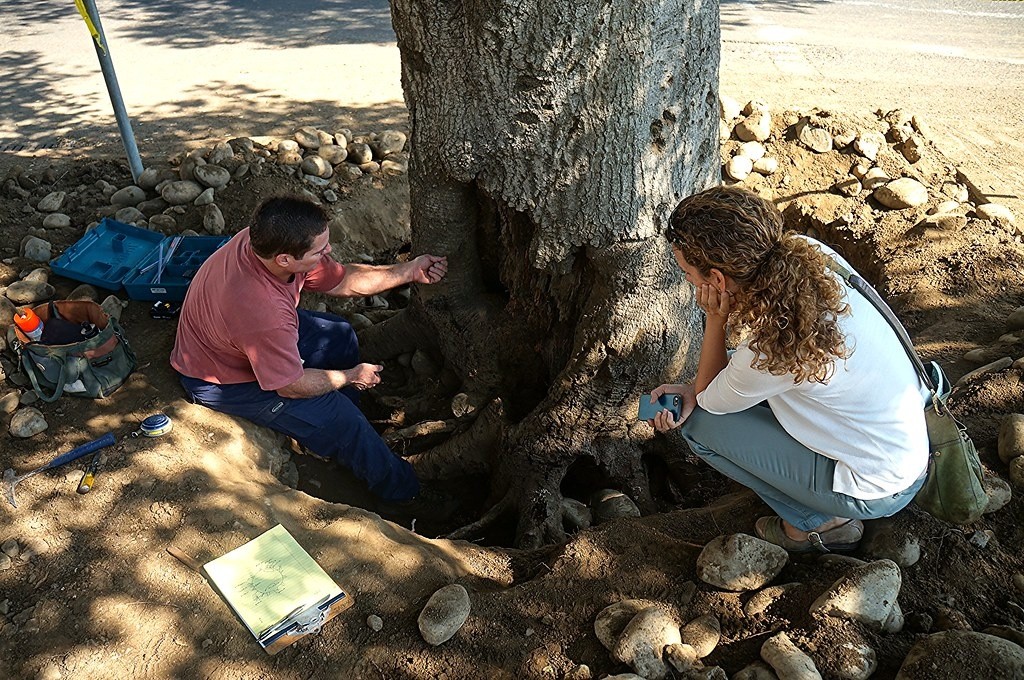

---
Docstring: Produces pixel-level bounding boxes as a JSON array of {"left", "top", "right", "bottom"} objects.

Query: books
[{"left": 204, "top": 523, "right": 346, "bottom": 649}]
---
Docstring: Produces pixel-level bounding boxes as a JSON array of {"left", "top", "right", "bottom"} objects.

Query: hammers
[{"left": 2, "top": 432, "right": 117, "bottom": 510}]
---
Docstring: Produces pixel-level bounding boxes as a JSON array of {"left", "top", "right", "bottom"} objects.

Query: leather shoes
[{"left": 755, "top": 516, "right": 865, "bottom": 553}]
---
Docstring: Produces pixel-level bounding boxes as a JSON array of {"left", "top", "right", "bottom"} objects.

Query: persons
[
  {"left": 648, "top": 187, "right": 931, "bottom": 551},
  {"left": 170, "top": 199, "right": 455, "bottom": 510}
]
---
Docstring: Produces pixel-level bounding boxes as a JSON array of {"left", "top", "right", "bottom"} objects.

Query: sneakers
[{"left": 395, "top": 491, "right": 450, "bottom": 522}]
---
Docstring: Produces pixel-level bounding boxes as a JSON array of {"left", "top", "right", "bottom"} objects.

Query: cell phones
[{"left": 638, "top": 392, "right": 682, "bottom": 423}]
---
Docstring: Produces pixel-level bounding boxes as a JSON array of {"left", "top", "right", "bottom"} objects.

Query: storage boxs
[{"left": 51, "top": 217, "right": 233, "bottom": 305}]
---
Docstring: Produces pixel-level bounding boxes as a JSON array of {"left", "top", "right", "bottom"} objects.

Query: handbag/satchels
[
  {"left": 913, "top": 361, "right": 991, "bottom": 526},
  {"left": 16, "top": 299, "right": 137, "bottom": 403}
]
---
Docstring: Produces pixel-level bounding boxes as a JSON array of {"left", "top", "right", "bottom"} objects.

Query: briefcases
[{"left": 49, "top": 216, "right": 233, "bottom": 303}]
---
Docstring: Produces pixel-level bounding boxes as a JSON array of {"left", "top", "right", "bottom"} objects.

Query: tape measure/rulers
[{"left": 132, "top": 414, "right": 172, "bottom": 438}]
